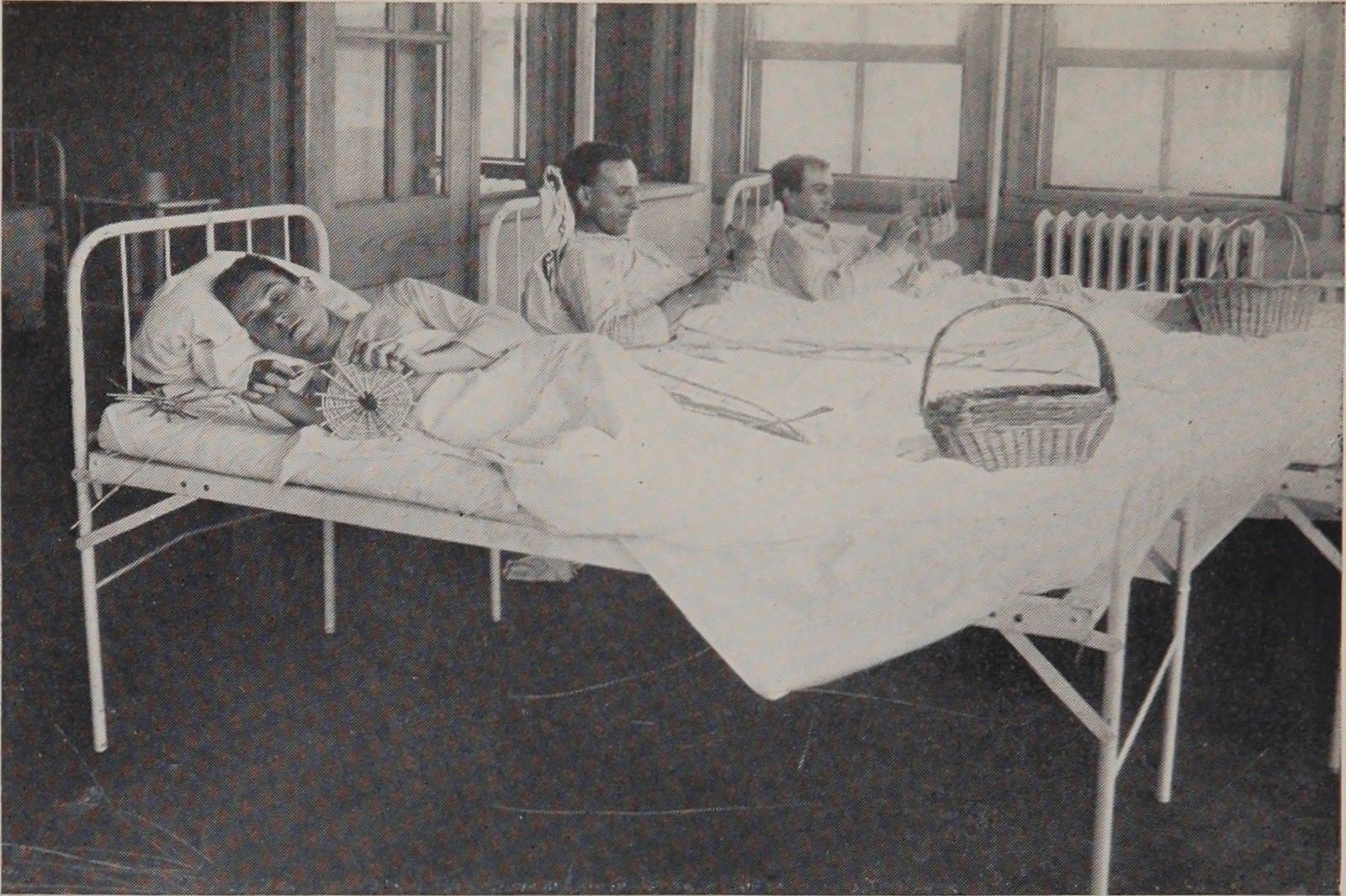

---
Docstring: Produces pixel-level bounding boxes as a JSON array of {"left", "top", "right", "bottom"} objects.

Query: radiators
[{"left": 1032, "top": 208, "right": 1264, "bottom": 295}]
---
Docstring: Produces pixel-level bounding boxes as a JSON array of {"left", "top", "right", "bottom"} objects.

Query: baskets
[
  {"left": 919, "top": 297, "right": 1118, "bottom": 468},
  {"left": 1179, "top": 211, "right": 1320, "bottom": 336}
]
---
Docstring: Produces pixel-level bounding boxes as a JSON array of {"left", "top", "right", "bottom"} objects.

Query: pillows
[
  {"left": 528, "top": 167, "right": 580, "bottom": 327},
  {"left": 126, "top": 251, "right": 370, "bottom": 387},
  {"left": 739, "top": 202, "right": 784, "bottom": 287}
]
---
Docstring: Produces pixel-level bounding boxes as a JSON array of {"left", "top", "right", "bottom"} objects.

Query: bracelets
[{"left": 682, "top": 288, "right": 699, "bottom": 305}]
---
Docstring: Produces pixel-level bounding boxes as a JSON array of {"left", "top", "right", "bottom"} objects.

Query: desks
[{"left": 72, "top": 192, "right": 220, "bottom": 310}]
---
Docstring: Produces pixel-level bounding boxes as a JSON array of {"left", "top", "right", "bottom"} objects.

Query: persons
[
  {"left": 553, "top": 143, "right": 973, "bottom": 355},
  {"left": 765, "top": 157, "right": 1062, "bottom": 319},
  {"left": 213, "top": 253, "right": 831, "bottom": 528}
]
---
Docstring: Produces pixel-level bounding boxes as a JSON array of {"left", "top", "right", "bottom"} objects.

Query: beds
[{"left": 1, "top": 122, "right": 1346, "bottom": 896}]
